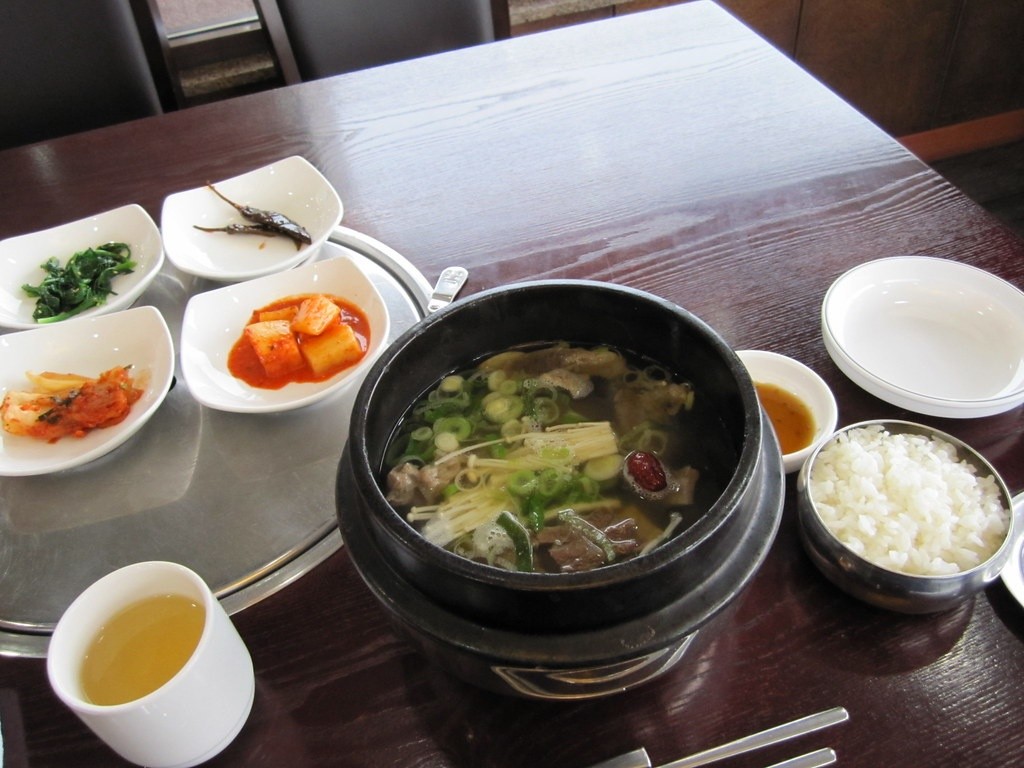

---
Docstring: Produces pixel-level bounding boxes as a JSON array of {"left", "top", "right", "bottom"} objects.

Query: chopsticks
[{"left": 650, "top": 707, "right": 850, "bottom": 768}]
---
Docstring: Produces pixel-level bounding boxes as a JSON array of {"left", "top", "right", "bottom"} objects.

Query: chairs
[
  {"left": 0, "top": 0, "right": 185, "bottom": 150},
  {"left": 252, "top": 0, "right": 512, "bottom": 86}
]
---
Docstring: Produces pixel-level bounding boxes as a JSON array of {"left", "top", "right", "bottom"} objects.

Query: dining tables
[{"left": 0, "top": 0, "right": 1023, "bottom": 768}]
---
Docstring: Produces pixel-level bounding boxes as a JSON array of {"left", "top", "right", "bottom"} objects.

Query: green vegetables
[{"left": 21, "top": 242, "right": 137, "bottom": 323}]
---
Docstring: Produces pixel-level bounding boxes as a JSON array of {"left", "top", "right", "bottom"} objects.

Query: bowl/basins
[
  {"left": 794, "top": 419, "right": 1014, "bottom": 614},
  {"left": 734, "top": 348, "right": 839, "bottom": 475},
  {"left": 333, "top": 279, "right": 786, "bottom": 704}
]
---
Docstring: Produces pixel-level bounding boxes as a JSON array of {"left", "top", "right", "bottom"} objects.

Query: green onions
[{"left": 390, "top": 366, "right": 694, "bottom": 572}]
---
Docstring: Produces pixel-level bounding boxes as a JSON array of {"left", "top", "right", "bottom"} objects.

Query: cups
[{"left": 46, "top": 561, "right": 255, "bottom": 768}]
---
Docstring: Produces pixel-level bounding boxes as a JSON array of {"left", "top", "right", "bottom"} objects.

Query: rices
[{"left": 808, "top": 424, "right": 1011, "bottom": 576}]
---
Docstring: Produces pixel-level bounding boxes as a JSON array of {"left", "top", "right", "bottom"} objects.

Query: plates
[
  {"left": 160, "top": 155, "right": 344, "bottom": 284},
  {"left": 0, "top": 304, "right": 175, "bottom": 476},
  {"left": 179, "top": 255, "right": 390, "bottom": 413},
  {"left": 822, "top": 256, "right": 1024, "bottom": 419},
  {"left": 0, "top": 203, "right": 165, "bottom": 330},
  {"left": 998, "top": 490, "right": 1024, "bottom": 608}
]
